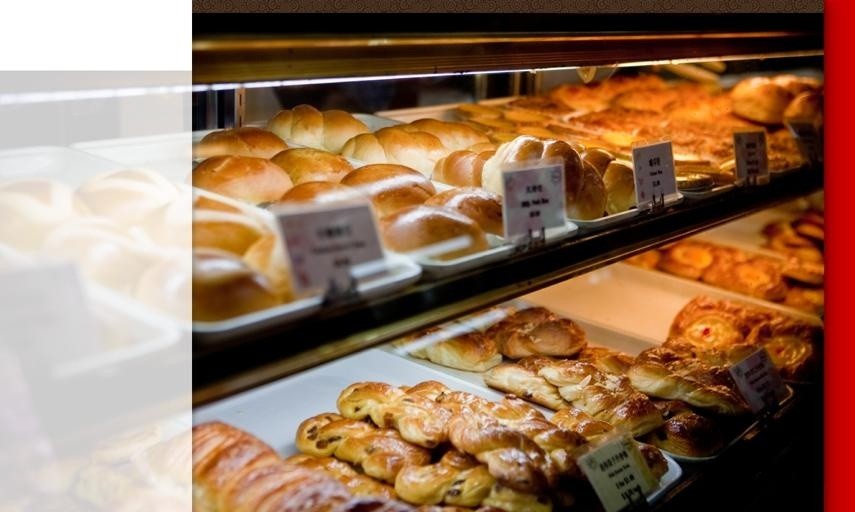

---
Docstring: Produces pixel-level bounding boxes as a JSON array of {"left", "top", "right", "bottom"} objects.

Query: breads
[{"left": 0, "top": 73, "right": 824, "bottom": 512}]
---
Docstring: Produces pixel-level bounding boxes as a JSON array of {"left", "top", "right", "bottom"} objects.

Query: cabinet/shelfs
[{"left": 194, "top": 0, "right": 826, "bottom": 510}]
